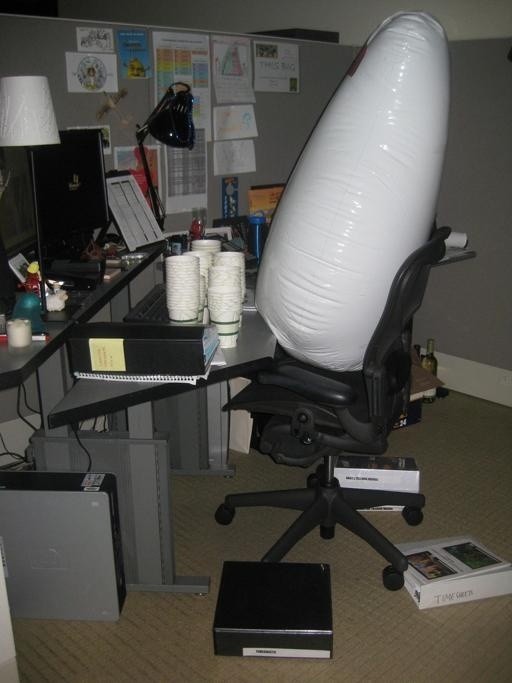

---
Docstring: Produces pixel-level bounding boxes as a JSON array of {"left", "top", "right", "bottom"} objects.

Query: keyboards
[{"left": 122, "top": 283, "right": 168, "bottom": 322}]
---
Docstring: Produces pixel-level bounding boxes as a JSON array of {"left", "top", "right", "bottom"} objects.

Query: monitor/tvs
[{"left": 26, "top": 129, "right": 110, "bottom": 272}]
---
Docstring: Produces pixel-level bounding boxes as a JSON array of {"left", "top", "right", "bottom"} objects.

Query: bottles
[
  {"left": 247, "top": 209, "right": 267, "bottom": 265},
  {"left": 413, "top": 336, "right": 437, "bottom": 402}
]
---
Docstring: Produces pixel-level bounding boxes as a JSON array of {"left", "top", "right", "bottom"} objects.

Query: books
[
  {"left": 68, "top": 349, "right": 231, "bottom": 387},
  {"left": 70, "top": 318, "right": 221, "bottom": 372}
]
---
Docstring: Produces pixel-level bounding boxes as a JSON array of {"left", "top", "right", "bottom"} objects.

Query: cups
[
  {"left": 191, "top": 206, "right": 208, "bottom": 238},
  {"left": 164, "top": 239, "right": 246, "bottom": 348},
  {"left": 6, "top": 319, "right": 33, "bottom": 353}
]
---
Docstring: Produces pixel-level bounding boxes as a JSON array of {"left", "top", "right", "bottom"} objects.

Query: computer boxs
[{"left": 0, "top": 468, "right": 127, "bottom": 623}]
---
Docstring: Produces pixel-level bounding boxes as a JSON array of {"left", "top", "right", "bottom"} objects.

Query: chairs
[{"left": 213, "top": 224, "right": 451, "bottom": 590}]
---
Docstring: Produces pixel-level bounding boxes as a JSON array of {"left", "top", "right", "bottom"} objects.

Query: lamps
[
  {"left": 135, "top": 81, "right": 197, "bottom": 233},
  {"left": 1, "top": 74, "right": 84, "bottom": 323}
]
